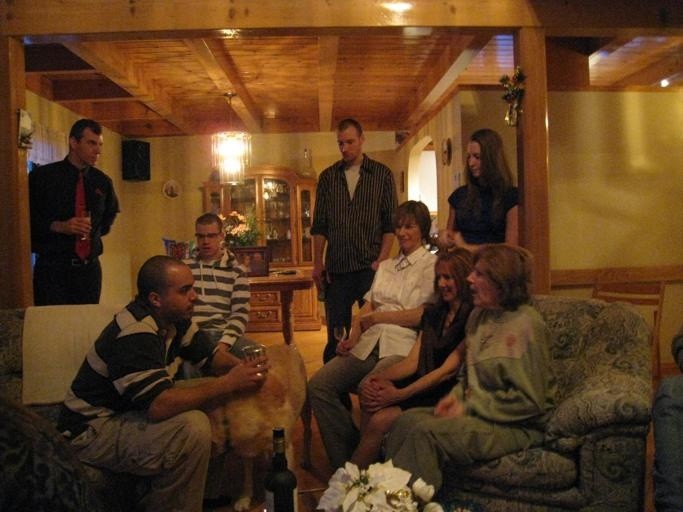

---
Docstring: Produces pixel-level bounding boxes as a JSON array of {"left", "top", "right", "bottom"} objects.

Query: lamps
[{"left": 209, "top": 90, "right": 256, "bottom": 186}]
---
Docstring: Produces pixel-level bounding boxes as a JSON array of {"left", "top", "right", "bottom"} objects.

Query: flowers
[
  {"left": 314, "top": 455, "right": 452, "bottom": 512},
  {"left": 212, "top": 208, "right": 270, "bottom": 247}
]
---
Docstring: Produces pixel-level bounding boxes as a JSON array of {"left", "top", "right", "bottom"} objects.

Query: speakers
[{"left": 122, "top": 140, "right": 150, "bottom": 180}]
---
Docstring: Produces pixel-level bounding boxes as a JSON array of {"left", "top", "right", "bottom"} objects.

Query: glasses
[{"left": 195, "top": 232, "right": 218, "bottom": 239}]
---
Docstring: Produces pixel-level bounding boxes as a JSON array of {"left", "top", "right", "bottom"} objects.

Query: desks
[{"left": 235, "top": 272, "right": 314, "bottom": 347}]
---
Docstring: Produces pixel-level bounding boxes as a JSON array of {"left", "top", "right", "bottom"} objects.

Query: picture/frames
[{"left": 230, "top": 245, "right": 269, "bottom": 276}]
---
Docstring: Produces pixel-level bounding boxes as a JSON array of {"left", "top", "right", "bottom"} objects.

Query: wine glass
[
  {"left": 79, "top": 209, "right": 92, "bottom": 242},
  {"left": 335, "top": 327, "right": 350, "bottom": 357}
]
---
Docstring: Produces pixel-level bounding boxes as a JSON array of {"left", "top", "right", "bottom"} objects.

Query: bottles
[
  {"left": 299, "top": 149, "right": 315, "bottom": 178},
  {"left": 316, "top": 269, "right": 326, "bottom": 301},
  {"left": 272, "top": 229, "right": 277, "bottom": 240},
  {"left": 263, "top": 427, "right": 298, "bottom": 512},
  {"left": 286, "top": 229, "right": 290, "bottom": 240},
  {"left": 269, "top": 201, "right": 284, "bottom": 220}
]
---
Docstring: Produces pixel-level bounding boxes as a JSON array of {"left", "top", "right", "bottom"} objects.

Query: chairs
[
  {"left": 0, "top": 304, "right": 268, "bottom": 511},
  {"left": 587, "top": 276, "right": 666, "bottom": 375},
  {"left": 434, "top": 293, "right": 657, "bottom": 511}
]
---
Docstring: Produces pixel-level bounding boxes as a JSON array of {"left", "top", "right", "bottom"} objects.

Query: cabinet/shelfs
[
  {"left": 204, "top": 179, "right": 225, "bottom": 218},
  {"left": 243, "top": 288, "right": 278, "bottom": 333},
  {"left": 289, "top": 270, "right": 320, "bottom": 332},
  {"left": 293, "top": 177, "right": 313, "bottom": 267},
  {"left": 224, "top": 165, "right": 291, "bottom": 266}
]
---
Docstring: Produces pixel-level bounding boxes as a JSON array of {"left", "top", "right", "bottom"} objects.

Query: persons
[
  {"left": 439, "top": 128, "right": 519, "bottom": 251},
  {"left": 306, "top": 199, "right": 438, "bottom": 468},
  {"left": 308, "top": 118, "right": 398, "bottom": 364},
  {"left": 650, "top": 325, "right": 682, "bottom": 511},
  {"left": 251, "top": 252, "right": 266, "bottom": 276},
  {"left": 238, "top": 255, "right": 252, "bottom": 272},
  {"left": 182, "top": 213, "right": 267, "bottom": 378},
  {"left": 26, "top": 116, "right": 120, "bottom": 306},
  {"left": 56, "top": 255, "right": 272, "bottom": 511},
  {"left": 342, "top": 247, "right": 478, "bottom": 471},
  {"left": 383, "top": 242, "right": 557, "bottom": 492}
]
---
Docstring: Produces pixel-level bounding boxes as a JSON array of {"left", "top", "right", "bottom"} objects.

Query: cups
[{"left": 241, "top": 344, "right": 271, "bottom": 381}]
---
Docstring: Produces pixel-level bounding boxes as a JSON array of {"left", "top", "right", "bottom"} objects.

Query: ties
[{"left": 74, "top": 170, "right": 92, "bottom": 263}]
